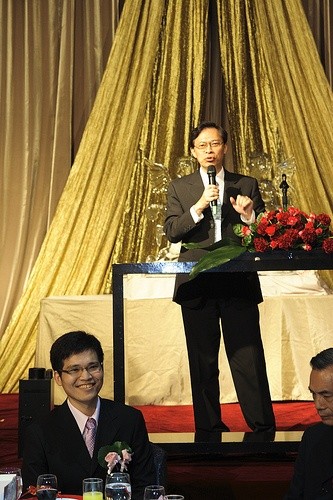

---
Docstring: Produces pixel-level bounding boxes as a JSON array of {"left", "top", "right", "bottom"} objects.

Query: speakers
[{"left": 18, "top": 379, "right": 55, "bottom": 459}]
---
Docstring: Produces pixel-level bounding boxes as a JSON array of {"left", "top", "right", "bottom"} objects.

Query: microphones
[{"left": 207, "top": 165, "right": 217, "bottom": 206}]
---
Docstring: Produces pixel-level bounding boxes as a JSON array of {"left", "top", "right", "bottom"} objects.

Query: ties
[
  {"left": 85, "top": 418, "right": 97, "bottom": 458},
  {"left": 211, "top": 199, "right": 221, "bottom": 220}
]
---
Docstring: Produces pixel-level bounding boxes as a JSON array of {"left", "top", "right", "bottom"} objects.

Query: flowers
[
  {"left": 96, "top": 440, "right": 134, "bottom": 475},
  {"left": 174, "top": 205, "right": 333, "bottom": 282}
]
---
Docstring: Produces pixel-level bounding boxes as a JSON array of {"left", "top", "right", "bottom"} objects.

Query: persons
[
  {"left": 288, "top": 347, "right": 333, "bottom": 500},
  {"left": 21, "top": 331, "right": 155, "bottom": 500},
  {"left": 161, "top": 122, "right": 288, "bottom": 431}
]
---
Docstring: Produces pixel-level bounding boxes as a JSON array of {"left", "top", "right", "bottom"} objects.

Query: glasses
[
  {"left": 195, "top": 141, "right": 223, "bottom": 149},
  {"left": 57, "top": 362, "right": 104, "bottom": 376}
]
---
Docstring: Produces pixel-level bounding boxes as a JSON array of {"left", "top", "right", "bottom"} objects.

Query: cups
[
  {"left": 83, "top": 478, "right": 103, "bottom": 500},
  {"left": 0, "top": 467, "right": 22, "bottom": 500},
  {"left": 36, "top": 474, "right": 58, "bottom": 500},
  {"left": 144, "top": 485, "right": 165, "bottom": 500},
  {"left": 105, "top": 473, "right": 131, "bottom": 500},
  {"left": 164, "top": 495, "right": 184, "bottom": 500}
]
